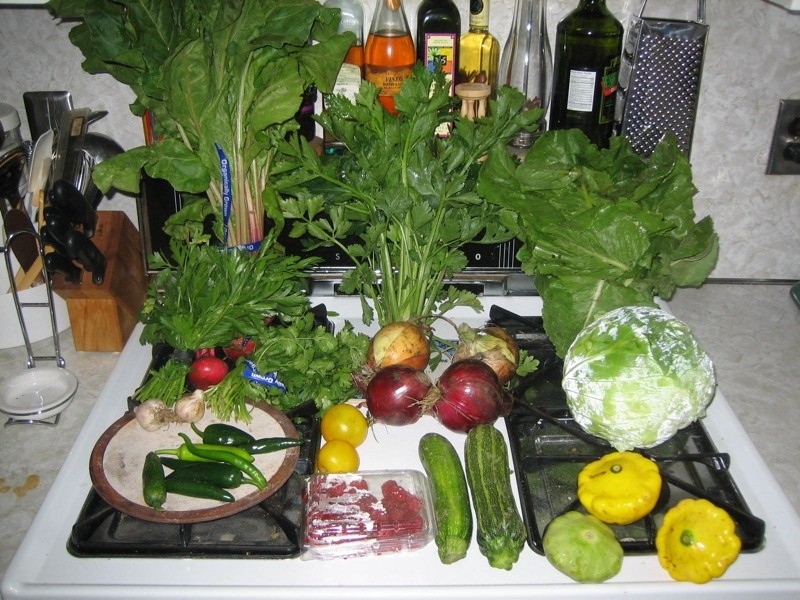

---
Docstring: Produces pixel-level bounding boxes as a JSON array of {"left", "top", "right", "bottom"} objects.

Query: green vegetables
[{"left": 46, "top": 1, "right": 720, "bottom": 428}]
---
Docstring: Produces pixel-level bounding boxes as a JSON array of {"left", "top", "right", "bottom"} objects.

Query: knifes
[{"left": 38, "top": 180, "right": 105, "bottom": 285}]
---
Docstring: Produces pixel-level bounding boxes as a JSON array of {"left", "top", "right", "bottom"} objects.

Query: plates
[
  {"left": 0, "top": 366, "right": 79, "bottom": 420},
  {"left": 90, "top": 392, "right": 302, "bottom": 525}
]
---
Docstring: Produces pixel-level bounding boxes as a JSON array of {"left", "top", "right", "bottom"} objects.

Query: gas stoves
[{"left": 0, "top": 162, "right": 798, "bottom": 600}]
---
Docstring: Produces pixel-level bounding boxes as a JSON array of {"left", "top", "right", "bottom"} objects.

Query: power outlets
[{"left": 764, "top": 98, "right": 800, "bottom": 176}]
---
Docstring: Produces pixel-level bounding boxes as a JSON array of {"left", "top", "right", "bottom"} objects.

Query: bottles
[
  {"left": 550, "top": 0, "right": 626, "bottom": 151},
  {"left": 459, "top": 1, "right": 500, "bottom": 118},
  {"left": 323, "top": 0, "right": 363, "bottom": 148},
  {"left": 364, "top": 1, "right": 417, "bottom": 116},
  {"left": 415, "top": 0, "right": 462, "bottom": 141}
]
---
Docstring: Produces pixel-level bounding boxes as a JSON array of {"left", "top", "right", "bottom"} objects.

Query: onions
[
  {"left": 414, "top": 362, "right": 509, "bottom": 431},
  {"left": 356, "top": 360, "right": 432, "bottom": 429}
]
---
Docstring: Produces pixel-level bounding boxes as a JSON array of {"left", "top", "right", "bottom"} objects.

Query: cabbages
[{"left": 564, "top": 303, "right": 724, "bottom": 449}]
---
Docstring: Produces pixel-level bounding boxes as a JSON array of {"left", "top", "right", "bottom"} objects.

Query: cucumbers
[
  {"left": 462, "top": 426, "right": 532, "bottom": 573},
  {"left": 422, "top": 416, "right": 472, "bottom": 564}
]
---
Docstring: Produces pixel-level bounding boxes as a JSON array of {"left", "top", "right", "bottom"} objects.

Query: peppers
[{"left": 132, "top": 422, "right": 307, "bottom": 513}]
